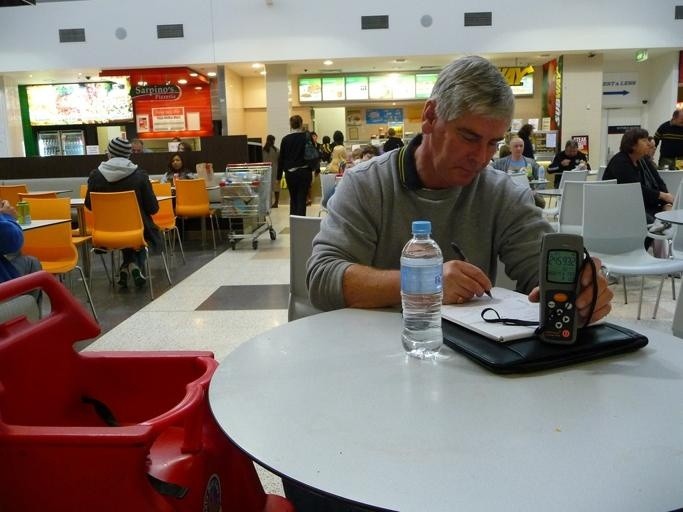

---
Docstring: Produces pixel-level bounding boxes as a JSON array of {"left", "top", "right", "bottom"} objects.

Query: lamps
[
  {"left": 630, "top": 48, "right": 649, "bottom": 64},
  {"left": 137, "top": 72, "right": 146, "bottom": 87}
]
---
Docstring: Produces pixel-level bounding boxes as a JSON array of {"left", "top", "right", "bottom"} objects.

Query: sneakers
[
  {"left": 128, "top": 262, "right": 147, "bottom": 288},
  {"left": 118, "top": 267, "right": 131, "bottom": 287},
  {"left": 272, "top": 201, "right": 278, "bottom": 208}
]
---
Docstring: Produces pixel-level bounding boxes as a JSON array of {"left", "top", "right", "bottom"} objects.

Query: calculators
[{"left": 539, "top": 234, "right": 584, "bottom": 345}]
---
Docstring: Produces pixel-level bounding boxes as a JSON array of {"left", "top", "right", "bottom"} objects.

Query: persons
[
  {"left": 129, "top": 138, "right": 153, "bottom": 154},
  {"left": 515, "top": 124, "right": 535, "bottom": 162},
  {"left": 547, "top": 140, "right": 591, "bottom": 189},
  {"left": 160, "top": 153, "right": 193, "bottom": 202},
  {"left": 176, "top": 143, "right": 192, "bottom": 152},
  {"left": 602, "top": 126, "right": 674, "bottom": 256},
  {"left": 304, "top": 55, "right": 613, "bottom": 328},
  {"left": 262, "top": 135, "right": 282, "bottom": 207},
  {"left": 83, "top": 136, "right": 158, "bottom": 289},
  {"left": 491, "top": 137, "right": 546, "bottom": 208},
  {"left": 499, "top": 132, "right": 515, "bottom": 158},
  {"left": 649, "top": 101, "right": 682, "bottom": 169},
  {"left": 645, "top": 137, "right": 660, "bottom": 171},
  {"left": 309, "top": 124, "right": 404, "bottom": 178},
  {"left": 274, "top": 116, "right": 317, "bottom": 217},
  {"left": 0, "top": 200, "right": 45, "bottom": 324}
]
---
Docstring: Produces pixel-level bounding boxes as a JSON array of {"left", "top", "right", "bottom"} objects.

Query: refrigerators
[{"left": 36, "top": 130, "right": 86, "bottom": 156}]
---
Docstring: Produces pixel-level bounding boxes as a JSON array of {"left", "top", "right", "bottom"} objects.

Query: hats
[{"left": 107, "top": 137, "right": 132, "bottom": 160}]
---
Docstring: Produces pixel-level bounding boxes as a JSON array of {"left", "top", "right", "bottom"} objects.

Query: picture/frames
[{"left": 349, "top": 128, "right": 359, "bottom": 140}]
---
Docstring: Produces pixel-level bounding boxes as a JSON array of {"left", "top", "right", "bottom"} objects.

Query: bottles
[
  {"left": 526, "top": 163, "right": 533, "bottom": 181},
  {"left": 16, "top": 201, "right": 30, "bottom": 225},
  {"left": 663, "top": 165, "right": 670, "bottom": 171},
  {"left": 41, "top": 135, "right": 83, "bottom": 154},
  {"left": 538, "top": 166, "right": 544, "bottom": 182},
  {"left": 397, "top": 219, "right": 445, "bottom": 360}
]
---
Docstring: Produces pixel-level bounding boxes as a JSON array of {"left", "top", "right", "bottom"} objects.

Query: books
[{"left": 433, "top": 280, "right": 540, "bottom": 343}]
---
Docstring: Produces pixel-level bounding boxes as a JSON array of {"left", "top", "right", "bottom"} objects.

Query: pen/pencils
[{"left": 451, "top": 242, "right": 493, "bottom": 299}]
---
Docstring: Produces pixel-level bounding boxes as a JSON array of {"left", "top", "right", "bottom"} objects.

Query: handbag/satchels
[{"left": 303, "top": 137, "right": 321, "bottom": 170}]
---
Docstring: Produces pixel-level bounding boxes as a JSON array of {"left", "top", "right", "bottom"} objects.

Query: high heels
[{"left": 305, "top": 200, "right": 312, "bottom": 207}]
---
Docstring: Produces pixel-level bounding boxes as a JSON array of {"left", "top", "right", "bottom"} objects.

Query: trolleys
[{"left": 218, "top": 161, "right": 279, "bottom": 253}]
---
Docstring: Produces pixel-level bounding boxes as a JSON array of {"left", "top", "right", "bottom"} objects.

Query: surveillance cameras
[
  {"left": 86, "top": 76, "right": 91, "bottom": 79},
  {"left": 304, "top": 69, "right": 309, "bottom": 72}
]
